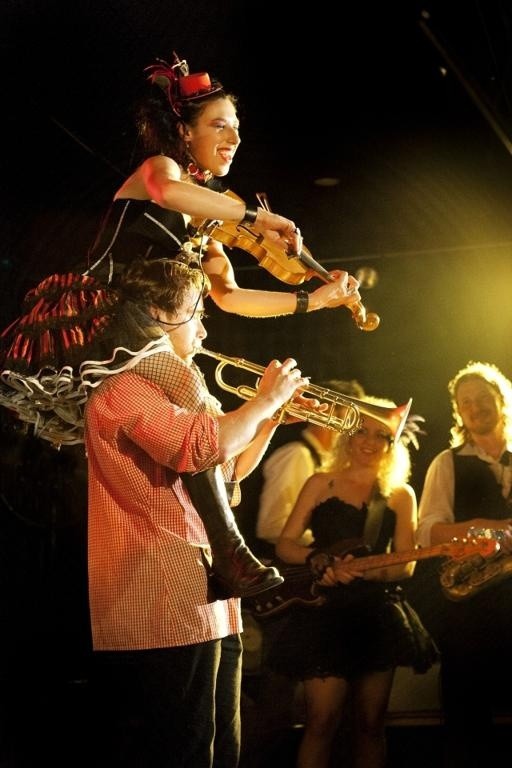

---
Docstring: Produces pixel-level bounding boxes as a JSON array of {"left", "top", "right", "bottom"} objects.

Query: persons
[
  {"left": 2, "top": 371, "right": 511, "bottom": 768},
  {"left": 0, "top": 74, "right": 360, "bottom": 604}
]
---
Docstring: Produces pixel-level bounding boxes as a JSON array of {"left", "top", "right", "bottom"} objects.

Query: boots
[{"left": 180, "top": 466, "right": 286, "bottom": 599}]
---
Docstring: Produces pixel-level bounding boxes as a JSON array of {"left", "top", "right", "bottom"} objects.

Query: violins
[{"left": 191, "top": 190, "right": 382, "bottom": 333}]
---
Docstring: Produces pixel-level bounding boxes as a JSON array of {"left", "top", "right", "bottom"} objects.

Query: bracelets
[
  {"left": 240, "top": 202, "right": 258, "bottom": 225},
  {"left": 295, "top": 290, "right": 309, "bottom": 314}
]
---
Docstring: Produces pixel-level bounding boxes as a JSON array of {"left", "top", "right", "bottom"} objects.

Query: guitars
[{"left": 240, "top": 537, "right": 499, "bottom": 619}]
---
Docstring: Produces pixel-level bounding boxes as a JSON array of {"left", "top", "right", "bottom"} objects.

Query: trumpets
[{"left": 195, "top": 347, "right": 413, "bottom": 445}]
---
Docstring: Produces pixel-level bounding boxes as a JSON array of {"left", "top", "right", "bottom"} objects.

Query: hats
[{"left": 170, "top": 71, "right": 221, "bottom": 104}]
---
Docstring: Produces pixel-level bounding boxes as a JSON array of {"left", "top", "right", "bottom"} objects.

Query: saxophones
[{"left": 432, "top": 529, "right": 512, "bottom": 604}]
